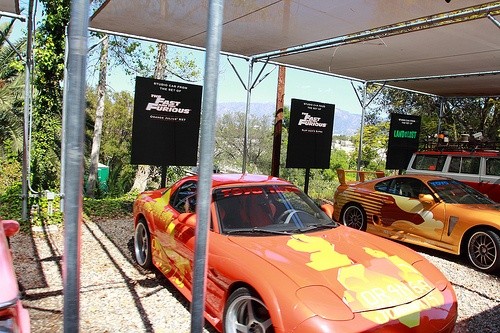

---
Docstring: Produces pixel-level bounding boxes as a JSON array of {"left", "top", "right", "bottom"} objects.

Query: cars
[
  {"left": 333, "top": 168, "right": 499, "bottom": 273},
  {"left": 0, "top": 218, "right": 32, "bottom": 333},
  {"left": 133, "top": 174, "right": 459, "bottom": 333}
]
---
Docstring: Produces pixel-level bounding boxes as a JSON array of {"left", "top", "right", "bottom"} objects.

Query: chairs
[{"left": 241, "top": 194, "right": 277, "bottom": 227}]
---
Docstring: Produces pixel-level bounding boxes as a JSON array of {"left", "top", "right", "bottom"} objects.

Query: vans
[{"left": 407, "top": 152, "right": 500, "bottom": 204}]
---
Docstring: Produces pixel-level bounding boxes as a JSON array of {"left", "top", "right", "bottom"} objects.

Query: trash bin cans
[{"left": 84, "top": 163, "right": 109, "bottom": 194}]
